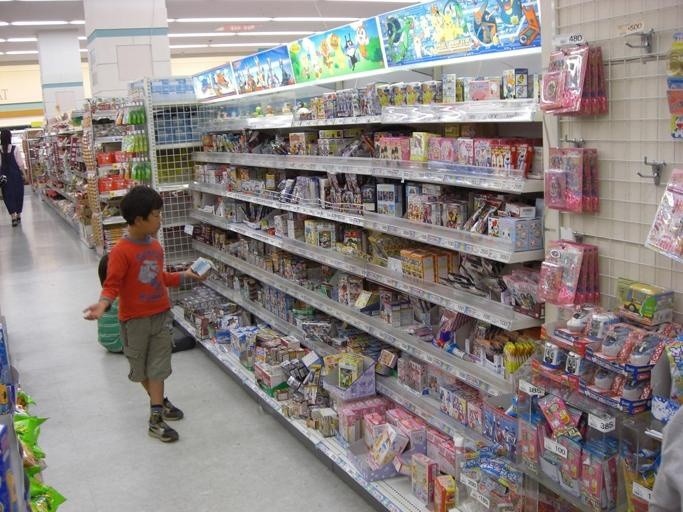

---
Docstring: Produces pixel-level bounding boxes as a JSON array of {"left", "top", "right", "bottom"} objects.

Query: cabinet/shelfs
[
  {"left": 23, "top": 97, "right": 151, "bottom": 258},
  {"left": 147, "top": 47, "right": 545, "bottom": 511},
  {"left": 519, "top": 320, "right": 674, "bottom": 510}
]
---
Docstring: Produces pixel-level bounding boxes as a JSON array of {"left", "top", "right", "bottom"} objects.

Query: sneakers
[
  {"left": 162, "top": 397, "right": 183, "bottom": 420},
  {"left": 149, "top": 421, "right": 179, "bottom": 442},
  {"left": 13, "top": 218, "right": 20, "bottom": 226}
]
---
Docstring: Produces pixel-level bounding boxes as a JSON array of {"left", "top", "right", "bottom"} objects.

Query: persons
[
  {"left": 0, "top": 129, "right": 28, "bottom": 227},
  {"left": 647, "top": 403, "right": 682, "bottom": 511},
  {"left": 98, "top": 255, "right": 197, "bottom": 354},
  {"left": 83, "top": 184, "right": 207, "bottom": 443}
]
